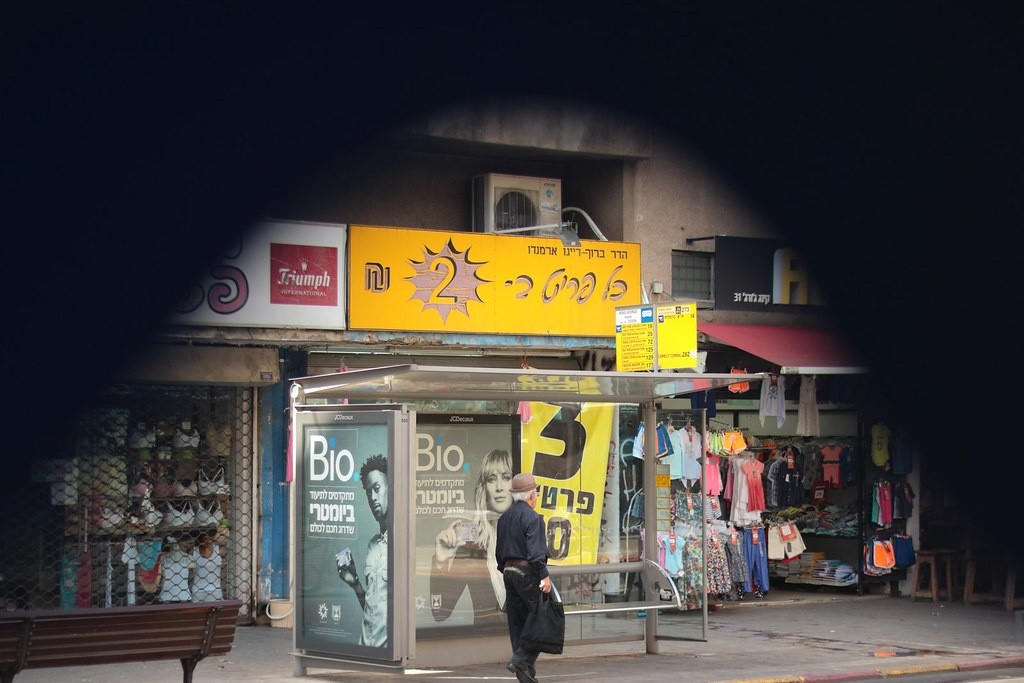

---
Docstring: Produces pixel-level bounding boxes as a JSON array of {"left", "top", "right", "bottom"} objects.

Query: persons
[
  {"left": 335, "top": 454, "right": 388, "bottom": 649},
  {"left": 494, "top": 473, "right": 565, "bottom": 683},
  {"left": 429, "top": 448, "right": 514, "bottom": 626},
  {"left": 158, "top": 538, "right": 228, "bottom": 605}
]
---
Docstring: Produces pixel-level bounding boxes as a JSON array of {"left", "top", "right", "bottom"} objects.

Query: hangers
[
  {"left": 744, "top": 523, "right": 766, "bottom": 531},
  {"left": 828, "top": 436, "right": 837, "bottom": 448},
  {"left": 709, "top": 420, "right": 749, "bottom": 433},
  {"left": 739, "top": 451, "right": 754, "bottom": 459},
  {"left": 769, "top": 517, "right": 796, "bottom": 528},
  {"left": 785, "top": 435, "right": 794, "bottom": 446},
  {"left": 667, "top": 417, "right": 674, "bottom": 425},
  {"left": 683, "top": 417, "right": 693, "bottom": 431},
  {"left": 679, "top": 520, "right": 727, "bottom": 535}
]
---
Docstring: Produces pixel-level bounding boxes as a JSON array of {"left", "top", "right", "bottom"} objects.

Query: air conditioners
[{"left": 471, "top": 172, "right": 562, "bottom": 238}]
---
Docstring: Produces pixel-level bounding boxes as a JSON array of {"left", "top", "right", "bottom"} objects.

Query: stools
[{"left": 910, "top": 549, "right": 957, "bottom": 604}]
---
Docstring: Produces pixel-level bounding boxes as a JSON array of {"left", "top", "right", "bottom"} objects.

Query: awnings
[{"left": 697, "top": 319, "right": 872, "bottom": 374}]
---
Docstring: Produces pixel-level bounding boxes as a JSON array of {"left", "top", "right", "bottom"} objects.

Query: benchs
[
  {"left": 0, "top": 599, "right": 242, "bottom": 683},
  {"left": 547, "top": 562, "right": 681, "bottom": 614}
]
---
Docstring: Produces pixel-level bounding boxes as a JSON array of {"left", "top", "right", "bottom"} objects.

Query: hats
[{"left": 508, "top": 473, "right": 539, "bottom": 492}]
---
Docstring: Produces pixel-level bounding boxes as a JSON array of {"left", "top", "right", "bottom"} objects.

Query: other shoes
[{"left": 506, "top": 662, "right": 538, "bottom": 683}]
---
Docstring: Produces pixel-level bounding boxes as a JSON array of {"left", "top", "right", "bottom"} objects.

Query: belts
[{"left": 504, "top": 558, "right": 529, "bottom": 567}]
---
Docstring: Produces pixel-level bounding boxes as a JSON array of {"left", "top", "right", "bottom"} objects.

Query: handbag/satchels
[{"left": 518, "top": 578, "right": 565, "bottom": 654}]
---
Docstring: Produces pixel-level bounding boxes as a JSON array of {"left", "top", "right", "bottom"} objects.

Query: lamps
[{"left": 496, "top": 221, "right": 582, "bottom": 247}]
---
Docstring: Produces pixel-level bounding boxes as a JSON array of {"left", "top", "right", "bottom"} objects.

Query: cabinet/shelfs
[
  {"left": 761, "top": 410, "right": 864, "bottom": 594},
  {"left": 40, "top": 399, "right": 232, "bottom": 609}
]
